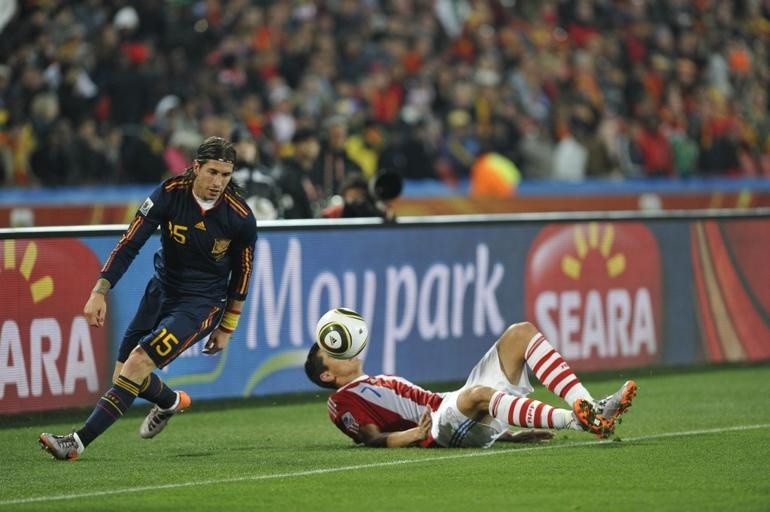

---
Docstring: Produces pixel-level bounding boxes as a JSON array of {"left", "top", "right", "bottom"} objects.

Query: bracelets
[{"left": 217, "top": 309, "right": 241, "bottom": 334}]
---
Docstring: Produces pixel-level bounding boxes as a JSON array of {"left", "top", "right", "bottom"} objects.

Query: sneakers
[
  {"left": 138, "top": 390, "right": 191, "bottom": 439},
  {"left": 38, "top": 430, "right": 78, "bottom": 460},
  {"left": 592, "top": 380, "right": 637, "bottom": 424},
  {"left": 571, "top": 397, "right": 613, "bottom": 438}
]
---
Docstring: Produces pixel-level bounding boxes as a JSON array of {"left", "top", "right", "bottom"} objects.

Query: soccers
[{"left": 316, "top": 307, "right": 368, "bottom": 359}]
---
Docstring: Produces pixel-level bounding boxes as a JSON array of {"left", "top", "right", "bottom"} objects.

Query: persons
[
  {"left": 2, "top": 2, "right": 769, "bottom": 220},
  {"left": 35, "top": 134, "right": 259, "bottom": 462},
  {"left": 303, "top": 319, "right": 637, "bottom": 450}
]
